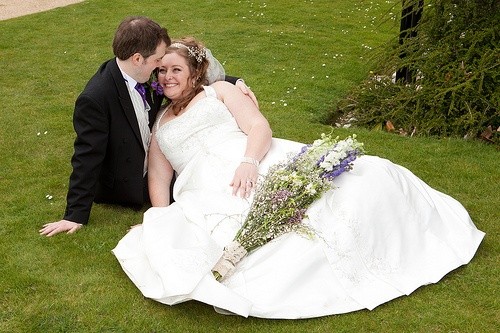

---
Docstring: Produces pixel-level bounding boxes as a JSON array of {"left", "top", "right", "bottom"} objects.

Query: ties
[{"left": 134, "top": 82, "right": 146, "bottom": 108}]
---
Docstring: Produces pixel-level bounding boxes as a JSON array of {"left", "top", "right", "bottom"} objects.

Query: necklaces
[{"left": 177, "top": 104, "right": 186, "bottom": 112}]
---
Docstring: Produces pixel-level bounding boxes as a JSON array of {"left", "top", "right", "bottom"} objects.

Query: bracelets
[{"left": 240, "top": 157, "right": 261, "bottom": 166}]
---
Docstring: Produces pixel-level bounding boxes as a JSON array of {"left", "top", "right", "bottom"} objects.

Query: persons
[
  {"left": 109, "top": 36, "right": 487, "bottom": 321},
  {"left": 38, "top": 17, "right": 259, "bottom": 237}
]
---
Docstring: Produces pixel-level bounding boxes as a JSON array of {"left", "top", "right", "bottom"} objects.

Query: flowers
[
  {"left": 211, "top": 126, "right": 370, "bottom": 283},
  {"left": 146, "top": 71, "right": 164, "bottom": 104},
  {"left": 159, "top": 97, "right": 172, "bottom": 110}
]
